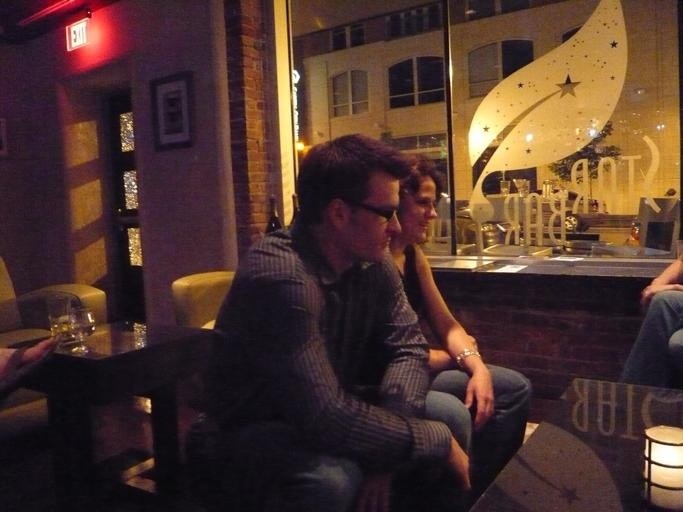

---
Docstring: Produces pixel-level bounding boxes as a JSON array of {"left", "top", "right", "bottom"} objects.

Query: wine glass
[{"left": 67, "top": 307, "right": 97, "bottom": 354}]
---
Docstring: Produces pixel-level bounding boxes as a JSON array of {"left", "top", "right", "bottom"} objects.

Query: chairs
[
  {"left": 1, "top": 257, "right": 108, "bottom": 441},
  {"left": 171, "top": 270, "right": 236, "bottom": 330}
]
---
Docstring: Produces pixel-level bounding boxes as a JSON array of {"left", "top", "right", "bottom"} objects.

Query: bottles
[
  {"left": 287, "top": 194, "right": 301, "bottom": 233},
  {"left": 264, "top": 197, "right": 282, "bottom": 238}
]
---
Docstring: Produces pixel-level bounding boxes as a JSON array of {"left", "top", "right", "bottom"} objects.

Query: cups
[{"left": 48, "top": 297, "right": 72, "bottom": 338}]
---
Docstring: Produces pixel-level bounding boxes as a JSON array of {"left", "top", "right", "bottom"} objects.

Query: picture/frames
[{"left": 149, "top": 70, "right": 196, "bottom": 153}]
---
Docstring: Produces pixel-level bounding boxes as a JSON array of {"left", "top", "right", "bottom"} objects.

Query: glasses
[{"left": 342, "top": 198, "right": 398, "bottom": 222}]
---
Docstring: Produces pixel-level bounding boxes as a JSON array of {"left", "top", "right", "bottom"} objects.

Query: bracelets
[{"left": 455, "top": 350, "right": 481, "bottom": 371}]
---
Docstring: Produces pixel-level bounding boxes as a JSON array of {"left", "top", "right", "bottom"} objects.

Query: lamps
[{"left": 642, "top": 425, "right": 683, "bottom": 512}]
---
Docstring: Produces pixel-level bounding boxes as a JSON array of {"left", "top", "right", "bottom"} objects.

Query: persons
[
  {"left": 390, "top": 152, "right": 532, "bottom": 489},
  {"left": 197, "top": 134, "right": 474, "bottom": 510},
  {"left": 617, "top": 254, "right": 683, "bottom": 385}
]
